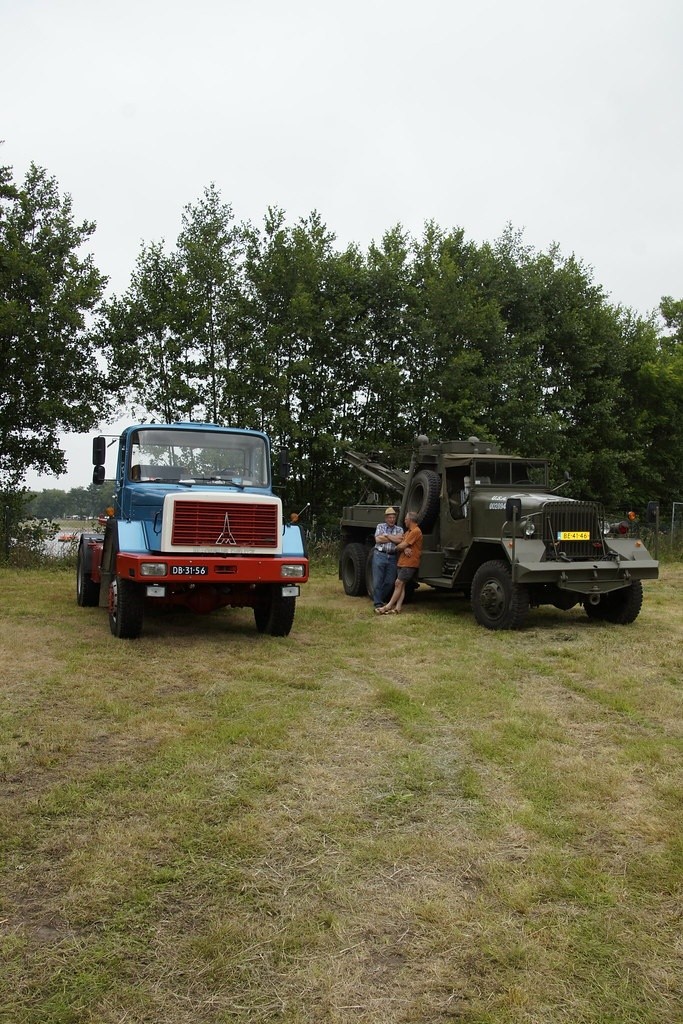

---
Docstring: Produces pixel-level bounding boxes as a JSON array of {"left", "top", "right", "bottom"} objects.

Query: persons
[
  {"left": 290, "top": 513, "right": 309, "bottom": 578},
  {"left": 372, "top": 507, "right": 422, "bottom": 615}
]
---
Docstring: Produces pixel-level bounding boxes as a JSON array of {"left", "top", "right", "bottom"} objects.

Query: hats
[{"left": 383, "top": 507, "right": 396, "bottom": 516}]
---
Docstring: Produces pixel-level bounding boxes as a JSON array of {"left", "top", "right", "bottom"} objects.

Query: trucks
[
  {"left": 336, "top": 432, "right": 661, "bottom": 631},
  {"left": 77, "top": 422, "right": 314, "bottom": 638}
]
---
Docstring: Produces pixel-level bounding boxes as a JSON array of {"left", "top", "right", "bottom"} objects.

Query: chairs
[
  {"left": 209, "top": 470, "right": 237, "bottom": 477},
  {"left": 450, "top": 490, "right": 462, "bottom": 518},
  {"left": 132, "top": 465, "right": 187, "bottom": 481}
]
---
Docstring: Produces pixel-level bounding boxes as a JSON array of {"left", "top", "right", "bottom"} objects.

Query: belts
[{"left": 375, "top": 548, "right": 396, "bottom": 555}]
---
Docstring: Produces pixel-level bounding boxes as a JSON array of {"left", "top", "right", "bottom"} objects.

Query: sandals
[
  {"left": 384, "top": 608, "right": 403, "bottom": 616},
  {"left": 373, "top": 604, "right": 390, "bottom": 615}
]
